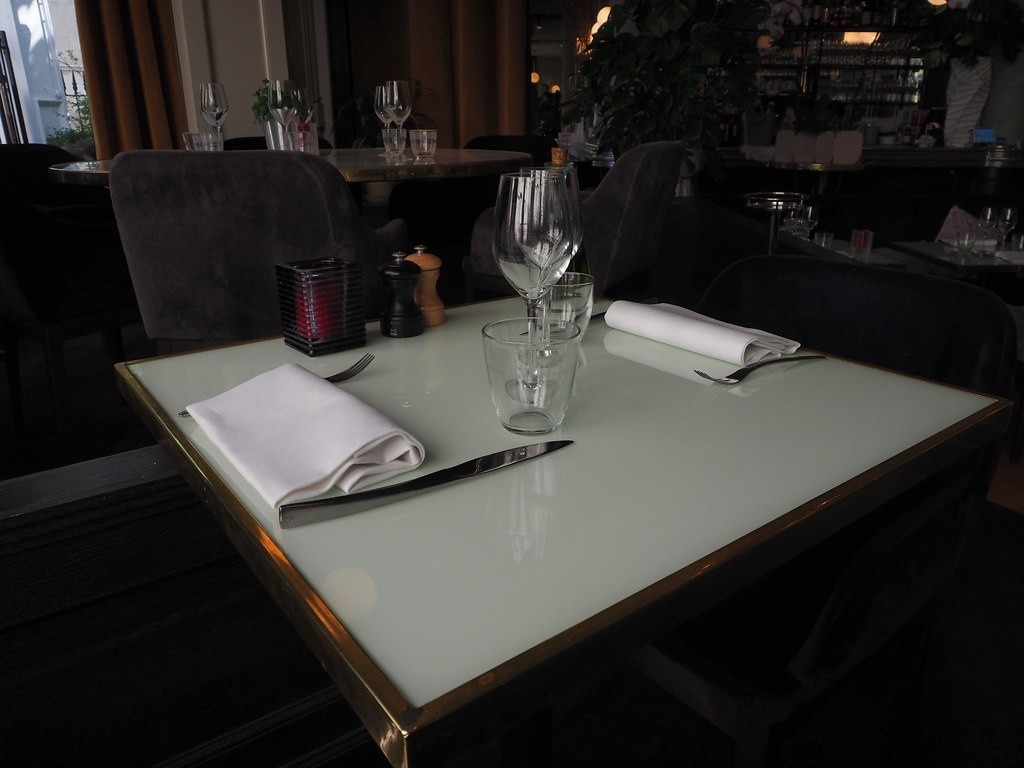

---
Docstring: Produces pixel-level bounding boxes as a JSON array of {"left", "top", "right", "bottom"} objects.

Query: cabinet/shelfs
[{"left": 706, "top": 26, "right": 940, "bottom": 136}]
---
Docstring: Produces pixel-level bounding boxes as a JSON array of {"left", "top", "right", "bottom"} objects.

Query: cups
[
  {"left": 264, "top": 120, "right": 319, "bottom": 156},
  {"left": 865, "top": 119, "right": 877, "bottom": 143},
  {"left": 548, "top": 270, "right": 594, "bottom": 330},
  {"left": 382, "top": 128, "right": 438, "bottom": 165},
  {"left": 815, "top": 231, "right": 834, "bottom": 249},
  {"left": 183, "top": 132, "right": 223, "bottom": 151},
  {"left": 957, "top": 232, "right": 973, "bottom": 252},
  {"left": 482, "top": 316, "right": 581, "bottom": 435},
  {"left": 879, "top": 119, "right": 895, "bottom": 145},
  {"left": 848, "top": 230, "right": 872, "bottom": 263}
]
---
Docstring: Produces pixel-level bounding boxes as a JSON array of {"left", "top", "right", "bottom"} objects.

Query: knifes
[{"left": 279, "top": 440, "right": 574, "bottom": 529}]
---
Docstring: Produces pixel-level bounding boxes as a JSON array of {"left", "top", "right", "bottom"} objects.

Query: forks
[
  {"left": 694, "top": 355, "right": 827, "bottom": 384},
  {"left": 178, "top": 353, "right": 375, "bottom": 416}
]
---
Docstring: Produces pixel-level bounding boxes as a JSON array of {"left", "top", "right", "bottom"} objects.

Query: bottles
[
  {"left": 902, "top": 124, "right": 912, "bottom": 145},
  {"left": 804, "top": 0, "right": 882, "bottom": 25}
]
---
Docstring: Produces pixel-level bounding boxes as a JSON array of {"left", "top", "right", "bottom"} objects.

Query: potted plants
[{"left": 252, "top": 78, "right": 308, "bottom": 150}]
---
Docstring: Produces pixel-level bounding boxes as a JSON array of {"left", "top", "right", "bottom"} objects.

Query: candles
[{"left": 274, "top": 256, "right": 367, "bottom": 356}]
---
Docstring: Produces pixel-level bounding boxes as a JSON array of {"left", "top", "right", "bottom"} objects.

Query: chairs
[
  {"left": 631, "top": 251, "right": 1017, "bottom": 768},
  {"left": 0, "top": 145, "right": 143, "bottom": 439},
  {"left": 474, "top": 136, "right": 715, "bottom": 301},
  {"left": 109, "top": 149, "right": 410, "bottom": 342}
]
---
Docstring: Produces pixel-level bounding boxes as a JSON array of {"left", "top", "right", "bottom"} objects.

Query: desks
[
  {"left": 52, "top": 146, "right": 540, "bottom": 186},
  {"left": 115, "top": 296, "right": 1024, "bottom": 768},
  {"left": 893, "top": 239, "right": 1024, "bottom": 277}
]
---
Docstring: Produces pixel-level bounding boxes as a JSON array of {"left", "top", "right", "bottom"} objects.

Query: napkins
[
  {"left": 604, "top": 300, "right": 802, "bottom": 368},
  {"left": 185, "top": 364, "right": 425, "bottom": 510}
]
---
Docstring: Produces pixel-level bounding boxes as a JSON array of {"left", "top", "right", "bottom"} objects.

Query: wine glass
[
  {"left": 199, "top": 83, "right": 228, "bottom": 134},
  {"left": 375, "top": 80, "right": 413, "bottom": 156},
  {"left": 977, "top": 206, "right": 1015, "bottom": 251},
  {"left": 783, "top": 204, "right": 817, "bottom": 242},
  {"left": 266, "top": 79, "right": 313, "bottom": 131},
  {"left": 491, "top": 166, "right": 584, "bottom": 403}
]
